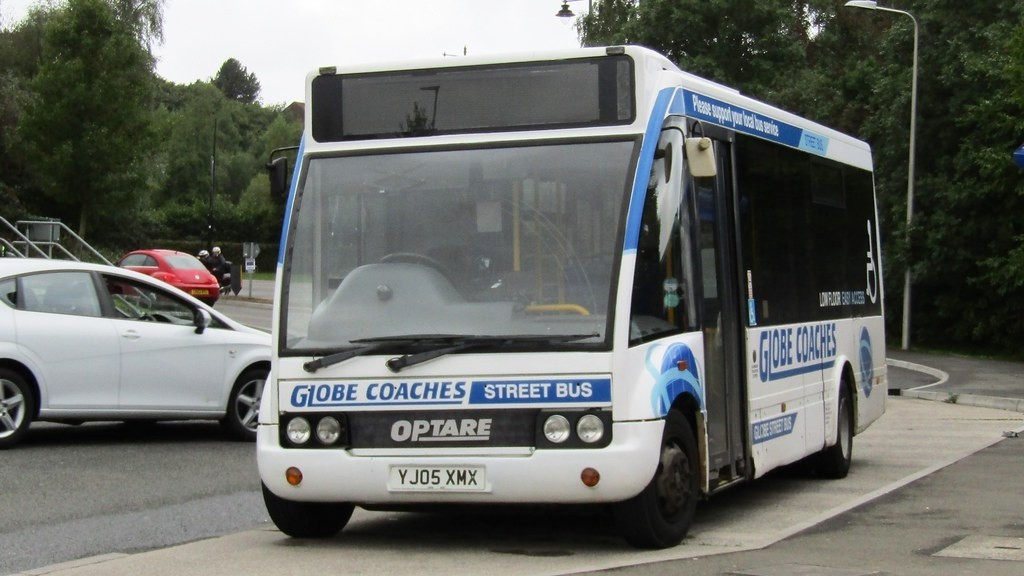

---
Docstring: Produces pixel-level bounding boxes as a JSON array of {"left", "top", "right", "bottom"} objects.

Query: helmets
[
  {"left": 212, "top": 247, "right": 221, "bottom": 256},
  {"left": 198, "top": 250, "right": 209, "bottom": 260}
]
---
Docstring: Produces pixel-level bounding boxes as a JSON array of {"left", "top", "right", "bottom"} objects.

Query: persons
[{"left": 198, "top": 247, "right": 228, "bottom": 288}]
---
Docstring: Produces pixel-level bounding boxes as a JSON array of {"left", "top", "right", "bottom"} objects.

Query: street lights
[
  {"left": 843, "top": 0, "right": 916, "bottom": 351},
  {"left": 556, "top": 0, "right": 600, "bottom": 49},
  {"left": 418, "top": 84, "right": 441, "bottom": 128}
]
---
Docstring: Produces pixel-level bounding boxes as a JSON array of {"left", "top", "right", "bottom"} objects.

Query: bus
[{"left": 254, "top": 42, "right": 889, "bottom": 553}]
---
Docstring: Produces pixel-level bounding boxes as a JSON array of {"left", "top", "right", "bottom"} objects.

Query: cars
[
  {"left": 113, "top": 248, "right": 221, "bottom": 305},
  {"left": 0, "top": 253, "right": 271, "bottom": 444}
]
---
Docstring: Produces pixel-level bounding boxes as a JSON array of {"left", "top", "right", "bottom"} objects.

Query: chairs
[
  {"left": 538, "top": 253, "right": 614, "bottom": 314},
  {"left": 20, "top": 278, "right": 82, "bottom": 317}
]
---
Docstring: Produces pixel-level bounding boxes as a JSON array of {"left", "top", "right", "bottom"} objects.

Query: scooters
[{"left": 203, "top": 262, "right": 235, "bottom": 297}]
